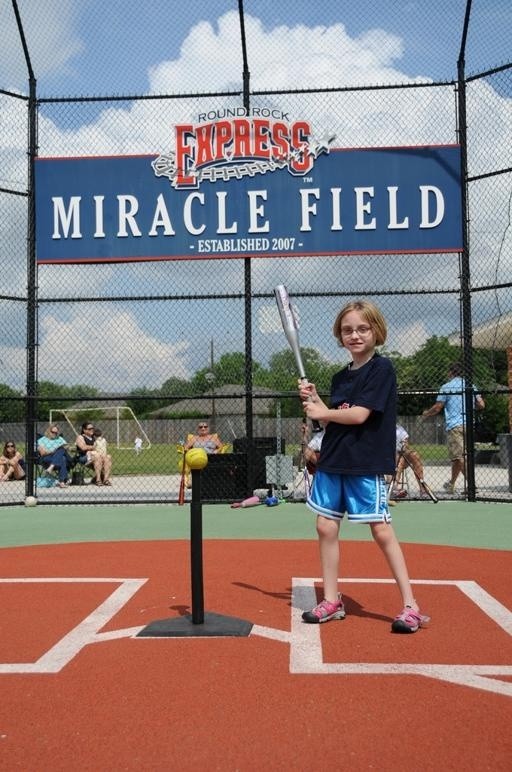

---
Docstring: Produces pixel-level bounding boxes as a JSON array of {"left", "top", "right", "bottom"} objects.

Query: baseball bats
[
  {"left": 398, "top": 450, "right": 438, "bottom": 504},
  {"left": 386, "top": 440, "right": 407, "bottom": 501},
  {"left": 274, "top": 284, "right": 325, "bottom": 433}
]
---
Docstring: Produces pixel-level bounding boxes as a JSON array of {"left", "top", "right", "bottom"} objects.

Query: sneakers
[
  {"left": 302, "top": 592, "right": 346, "bottom": 623},
  {"left": 391, "top": 487, "right": 408, "bottom": 498},
  {"left": 391, "top": 605, "right": 430, "bottom": 633},
  {"left": 443, "top": 481, "right": 455, "bottom": 494}
]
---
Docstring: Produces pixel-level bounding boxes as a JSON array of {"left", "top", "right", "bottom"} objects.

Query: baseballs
[
  {"left": 184, "top": 448, "right": 209, "bottom": 471},
  {"left": 24, "top": 496, "right": 37, "bottom": 508}
]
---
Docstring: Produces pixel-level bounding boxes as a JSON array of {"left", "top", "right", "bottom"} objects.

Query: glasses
[
  {"left": 340, "top": 327, "right": 371, "bottom": 335},
  {"left": 84, "top": 427, "right": 94, "bottom": 430},
  {"left": 50, "top": 430, "right": 58, "bottom": 435},
  {"left": 6, "top": 444, "right": 14, "bottom": 448},
  {"left": 198, "top": 425, "right": 209, "bottom": 429}
]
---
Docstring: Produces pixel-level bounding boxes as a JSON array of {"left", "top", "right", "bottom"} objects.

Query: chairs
[
  {"left": 76, "top": 444, "right": 105, "bottom": 485},
  {"left": 385, "top": 467, "right": 411, "bottom": 502},
  {"left": 177, "top": 433, "right": 231, "bottom": 488},
  {"left": 34, "top": 433, "right": 78, "bottom": 487}
]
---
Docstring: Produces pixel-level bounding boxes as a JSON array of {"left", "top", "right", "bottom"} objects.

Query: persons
[
  {"left": 76, "top": 424, "right": 112, "bottom": 486},
  {"left": 302, "top": 301, "right": 431, "bottom": 632},
  {"left": 135, "top": 435, "right": 143, "bottom": 456},
  {"left": 1, "top": 439, "right": 27, "bottom": 481},
  {"left": 302, "top": 414, "right": 426, "bottom": 498},
  {"left": 36, "top": 426, "right": 72, "bottom": 488},
  {"left": 183, "top": 422, "right": 223, "bottom": 455},
  {"left": 422, "top": 364, "right": 485, "bottom": 495}
]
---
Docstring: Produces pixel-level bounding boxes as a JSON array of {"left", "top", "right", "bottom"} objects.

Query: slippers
[{"left": 95, "top": 480, "right": 111, "bottom": 486}]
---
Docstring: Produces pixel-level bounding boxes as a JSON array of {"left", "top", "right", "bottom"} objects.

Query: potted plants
[{"left": 485, "top": 395, "right": 511, "bottom": 468}]
